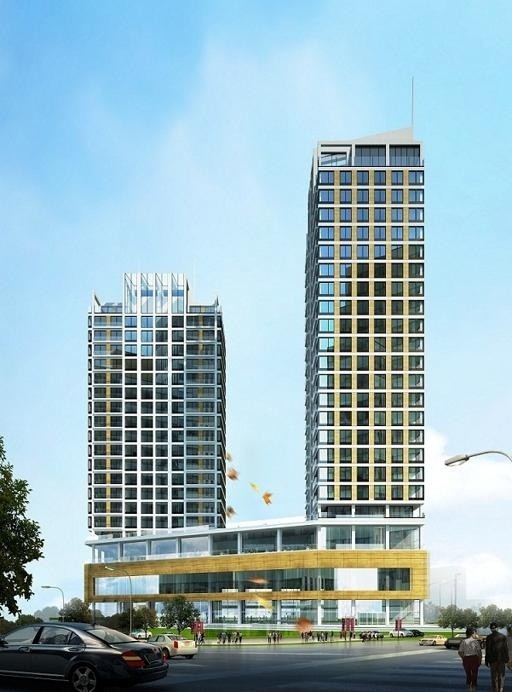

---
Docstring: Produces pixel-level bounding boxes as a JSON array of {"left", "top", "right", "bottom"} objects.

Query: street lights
[
  {"left": 105, "top": 566, "right": 133, "bottom": 635},
  {"left": 41, "top": 586, "right": 65, "bottom": 621}
]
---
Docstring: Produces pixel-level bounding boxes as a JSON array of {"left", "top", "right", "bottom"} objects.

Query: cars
[
  {"left": 130, "top": 629, "right": 152, "bottom": 640},
  {"left": 419, "top": 635, "right": 448, "bottom": 646},
  {"left": 409, "top": 630, "right": 424, "bottom": 637},
  {"left": 445, "top": 633, "right": 486, "bottom": 649},
  {"left": 148, "top": 634, "right": 198, "bottom": 660},
  {"left": 389, "top": 628, "right": 414, "bottom": 638},
  {"left": 0, "top": 623, "right": 169, "bottom": 691}
]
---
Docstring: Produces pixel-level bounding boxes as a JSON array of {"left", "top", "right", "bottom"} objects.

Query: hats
[{"left": 490, "top": 622, "right": 497, "bottom": 629}]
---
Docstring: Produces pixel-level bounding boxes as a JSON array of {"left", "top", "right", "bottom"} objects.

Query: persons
[
  {"left": 458, "top": 626, "right": 482, "bottom": 691},
  {"left": 340, "top": 630, "right": 355, "bottom": 637},
  {"left": 484, "top": 622, "right": 508, "bottom": 691},
  {"left": 194, "top": 632, "right": 204, "bottom": 644},
  {"left": 359, "top": 631, "right": 380, "bottom": 642},
  {"left": 266, "top": 630, "right": 283, "bottom": 645},
  {"left": 217, "top": 631, "right": 244, "bottom": 645},
  {"left": 504, "top": 623, "right": 512, "bottom": 673},
  {"left": 301, "top": 631, "right": 335, "bottom": 644}
]
